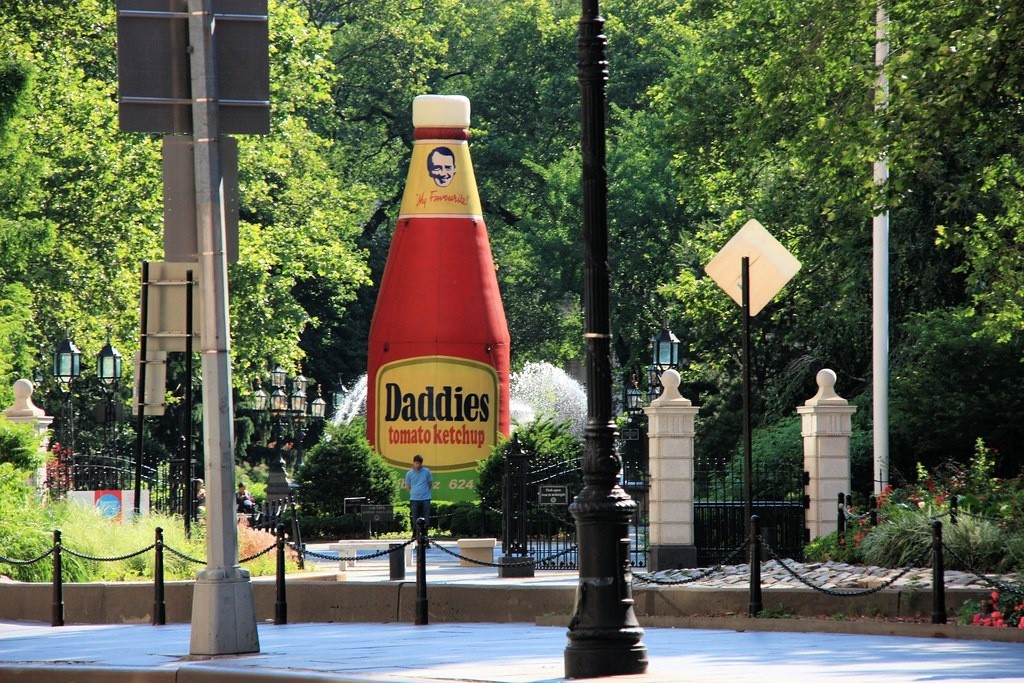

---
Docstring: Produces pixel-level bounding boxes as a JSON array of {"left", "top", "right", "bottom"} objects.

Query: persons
[
  {"left": 405, "top": 454, "right": 432, "bottom": 549},
  {"left": 235, "top": 482, "right": 254, "bottom": 513},
  {"left": 192, "top": 478, "right": 206, "bottom": 516}
]
